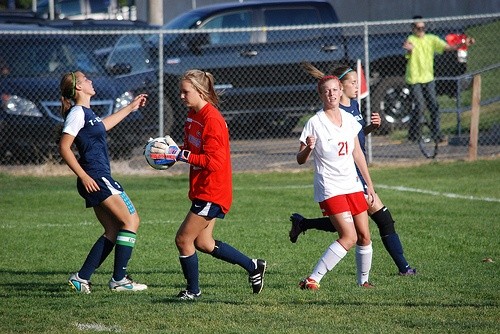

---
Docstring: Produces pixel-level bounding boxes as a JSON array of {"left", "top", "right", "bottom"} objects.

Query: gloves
[{"left": 149, "top": 135, "right": 191, "bottom": 164}]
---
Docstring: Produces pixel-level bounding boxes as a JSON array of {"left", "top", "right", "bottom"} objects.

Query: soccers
[{"left": 144, "top": 137, "right": 175, "bottom": 170}]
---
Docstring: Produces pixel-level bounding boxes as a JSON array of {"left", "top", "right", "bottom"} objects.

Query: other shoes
[
  {"left": 413, "top": 133, "right": 430, "bottom": 143},
  {"left": 431, "top": 134, "right": 442, "bottom": 142}
]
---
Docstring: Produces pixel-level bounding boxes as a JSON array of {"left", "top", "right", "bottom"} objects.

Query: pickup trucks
[{"left": 0, "top": 0, "right": 474, "bottom": 164}]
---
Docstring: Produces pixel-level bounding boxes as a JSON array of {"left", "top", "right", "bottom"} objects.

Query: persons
[
  {"left": 289, "top": 67, "right": 417, "bottom": 277},
  {"left": 59, "top": 69, "right": 148, "bottom": 294},
  {"left": 403, "top": 16, "right": 462, "bottom": 144},
  {"left": 150, "top": 69, "right": 266, "bottom": 299},
  {"left": 446, "top": 23, "right": 475, "bottom": 75},
  {"left": 297, "top": 59, "right": 377, "bottom": 290}
]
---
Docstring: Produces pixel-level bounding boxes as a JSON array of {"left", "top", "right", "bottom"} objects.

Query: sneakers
[
  {"left": 289, "top": 212, "right": 305, "bottom": 243},
  {"left": 177, "top": 290, "right": 202, "bottom": 298},
  {"left": 108, "top": 276, "right": 147, "bottom": 292},
  {"left": 399, "top": 268, "right": 418, "bottom": 275},
  {"left": 68, "top": 272, "right": 92, "bottom": 294},
  {"left": 249, "top": 258, "right": 266, "bottom": 294},
  {"left": 299, "top": 277, "right": 320, "bottom": 290},
  {"left": 362, "top": 282, "right": 374, "bottom": 288}
]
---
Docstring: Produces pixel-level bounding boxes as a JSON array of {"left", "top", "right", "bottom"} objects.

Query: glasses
[{"left": 414, "top": 25, "right": 426, "bottom": 31}]
